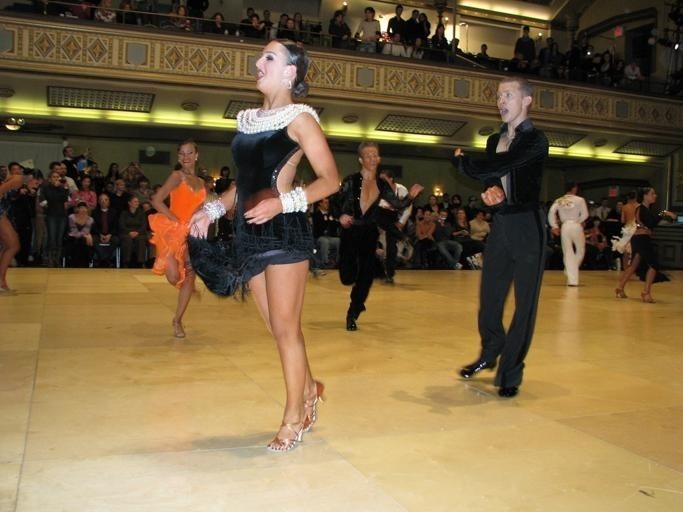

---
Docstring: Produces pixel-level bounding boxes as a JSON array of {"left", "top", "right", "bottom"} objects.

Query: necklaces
[{"left": 236, "top": 102, "right": 320, "bottom": 135}]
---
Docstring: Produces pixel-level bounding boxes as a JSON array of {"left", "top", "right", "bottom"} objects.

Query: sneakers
[{"left": 453, "top": 261, "right": 462, "bottom": 271}]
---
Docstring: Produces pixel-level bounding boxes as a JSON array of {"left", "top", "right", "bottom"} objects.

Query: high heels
[
  {"left": 615, "top": 287, "right": 628, "bottom": 299},
  {"left": 640, "top": 291, "right": 655, "bottom": 304},
  {"left": 302, "top": 380, "right": 325, "bottom": 434},
  {"left": 171, "top": 318, "right": 186, "bottom": 338},
  {"left": 267, "top": 407, "right": 309, "bottom": 452}
]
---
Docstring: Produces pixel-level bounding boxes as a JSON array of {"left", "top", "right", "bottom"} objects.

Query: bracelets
[
  {"left": 279, "top": 186, "right": 308, "bottom": 215},
  {"left": 201, "top": 198, "right": 227, "bottom": 223}
]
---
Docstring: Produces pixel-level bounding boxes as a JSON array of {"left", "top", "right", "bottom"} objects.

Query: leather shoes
[
  {"left": 345, "top": 319, "right": 357, "bottom": 331},
  {"left": 498, "top": 383, "right": 520, "bottom": 397},
  {"left": 461, "top": 358, "right": 497, "bottom": 379}
]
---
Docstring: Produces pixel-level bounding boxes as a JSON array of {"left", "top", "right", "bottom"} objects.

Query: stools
[{"left": 90, "top": 242, "right": 123, "bottom": 268}]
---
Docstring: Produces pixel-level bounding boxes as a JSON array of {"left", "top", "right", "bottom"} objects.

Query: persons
[
  {"left": 378, "top": 169, "right": 413, "bottom": 285},
  {"left": 0, "top": 146, "right": 162, "bottom": 292},
  {"left": 547, "top": 180, "right": 589, "bottom": 286},
  {"left": 382, "top": 4, "right": 449, "bottom": 61},
  {"left": 313, "top": 198, "right": 340, "bottom": 266},
  {"left": 452, "top": 76, "right": 549, "bottom": 398},
  {"left": 590, "top": 199, "right": 623, "bottom": 271},
  {"left": 354, "top": 7, "right": 381, "bottom": 54},
  {"left": 328, "top": 11, "right": 351, "bottom": 50},
  {"left": 337, "top": 142, "right": 425, "bottom": 331},
  {"left": 478, "top": 44, "right": 489, "bottom": 60},
  {"left": 615, "top": 187, "right": 677, "bottom": 304},
  {"left": 148, "top": 139, "right": 209, "bottom": 340},
  {"left": 95, "top": 1, "right": 306, "bottom": 43},
  {"left": 413, "top": 195, "right": 491, "bottom": 270},
  {"left": 515, "top": 24, "right": 641, "bottom": 92},
  {"left": 200, "top": 165, "right": 235, "bottom": 196},
  {"left": 186, "top": 41, "right": 340, "bottom": 452},
  {"left": 620, "top": 191, "right": 641, "bottom": 270}
]
